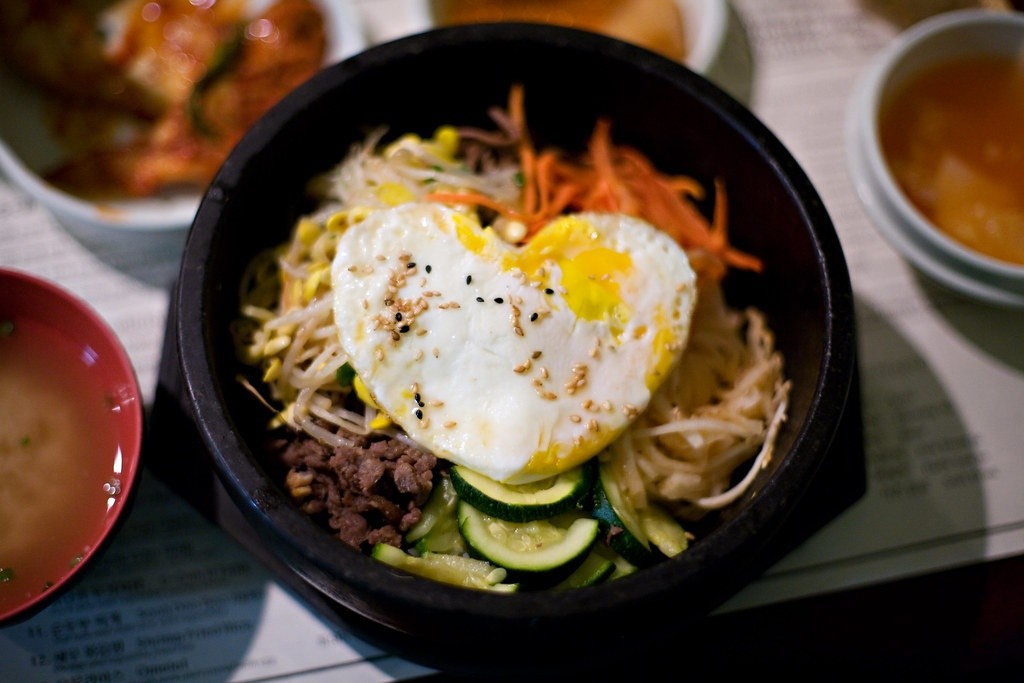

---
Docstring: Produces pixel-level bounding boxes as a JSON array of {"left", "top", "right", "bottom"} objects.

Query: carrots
[{"left": 418, "top": 82, "right": 763, "bottom": 276}]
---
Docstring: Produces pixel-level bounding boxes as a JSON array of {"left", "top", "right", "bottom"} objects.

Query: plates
[
  {"left": 334, "top": 0, "right": 730, "bottom": 102},
  {"left": 150, "top": 275, "right": 865, "bottom": 683}
]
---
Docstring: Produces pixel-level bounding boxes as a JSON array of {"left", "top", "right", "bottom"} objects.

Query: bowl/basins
[
  {"left": 0, "top": 0, "right": 340, "bottom": 231},
  {"left": 849, "top": 8, "right": 1024, "bottom": 311},
  {"left": 0, "top": 268, "right": 142, "bottom": 620},
  {"left": 179, "top": 20, "right": 855, "bottom": 650}
]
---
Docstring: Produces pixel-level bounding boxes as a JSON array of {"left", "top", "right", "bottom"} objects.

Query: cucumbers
[{"left": 367, "top": 448, "right": 690, "bottom": 593}]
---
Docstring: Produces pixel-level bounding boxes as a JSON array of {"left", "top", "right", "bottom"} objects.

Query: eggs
[{"left": 333, "top": 202, "right": 699, "bottom": 485}]
